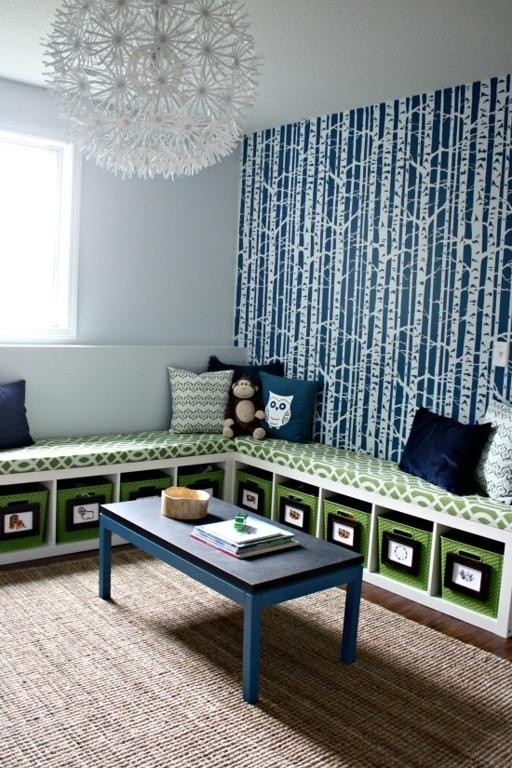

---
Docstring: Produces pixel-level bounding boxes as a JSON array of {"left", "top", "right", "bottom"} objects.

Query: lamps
[{"left": 40, "top": 0, "right": 265, "bottom": 182}]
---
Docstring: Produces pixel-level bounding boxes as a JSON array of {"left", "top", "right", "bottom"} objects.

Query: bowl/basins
[{"left": 162, "top": 486, "right": 210, "bottom": 519}]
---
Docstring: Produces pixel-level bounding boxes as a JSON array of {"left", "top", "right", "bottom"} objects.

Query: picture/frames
[
  {"left": 0, "top": 502, "right": 41, "bottom": 542},
  {"left": 278, "top": 495, "right": 311, "bottom": 534},
  {"left": 130, "top": 488, "right": 165, "bottom": 502},
  {"left": 326, "top": 512, "right": 362, "bottom": 553},
  {"left": 380, "top": 529, "right": 423, "bottom": 578},
  {"left": 444, "top": 551, "right": 492, "bottom": 603},
  {"left": 238, "top": 480, "right": 266, "bottom": 516},
  {"left": 66, "top": 495, "right": 105, "bottom": 533},
  {"left": 187, "top": 481, "right": 220, "bottom": 498}
]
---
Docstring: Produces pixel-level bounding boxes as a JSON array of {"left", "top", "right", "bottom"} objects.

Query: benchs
[
  {"left": 233, "top": 434, "right": 512, "bottom": 658},
  {"left": 0, "top": 336, "right": 246, "bottom": 570}
]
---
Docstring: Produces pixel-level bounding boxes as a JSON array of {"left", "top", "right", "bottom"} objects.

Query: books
[{"left": 190, "top": 517, "right": 299, "bottom": 559}]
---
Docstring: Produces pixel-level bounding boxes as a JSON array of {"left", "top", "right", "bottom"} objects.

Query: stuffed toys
[{"left": 222, "top": 377, "right": 266, "bottom": 439}]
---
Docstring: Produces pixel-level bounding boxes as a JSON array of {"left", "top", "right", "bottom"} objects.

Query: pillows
[
  {"left": 206, "top": 354, "right": 285, "bottom": 398},
  {"left": 0, "top": 379, "right": 35, "bottom": 451},
  {"left": 398, "top": 406, "right": 493, "bottom": 497},
  {"left": 257, "top": 370, "right": 317, "bottom": 443},
  {"left": 167, "top": 365, "right": 236, "bottom": 434},
  {"left": 472, "top": 399, "right": 512, "bottom": 505}
]
